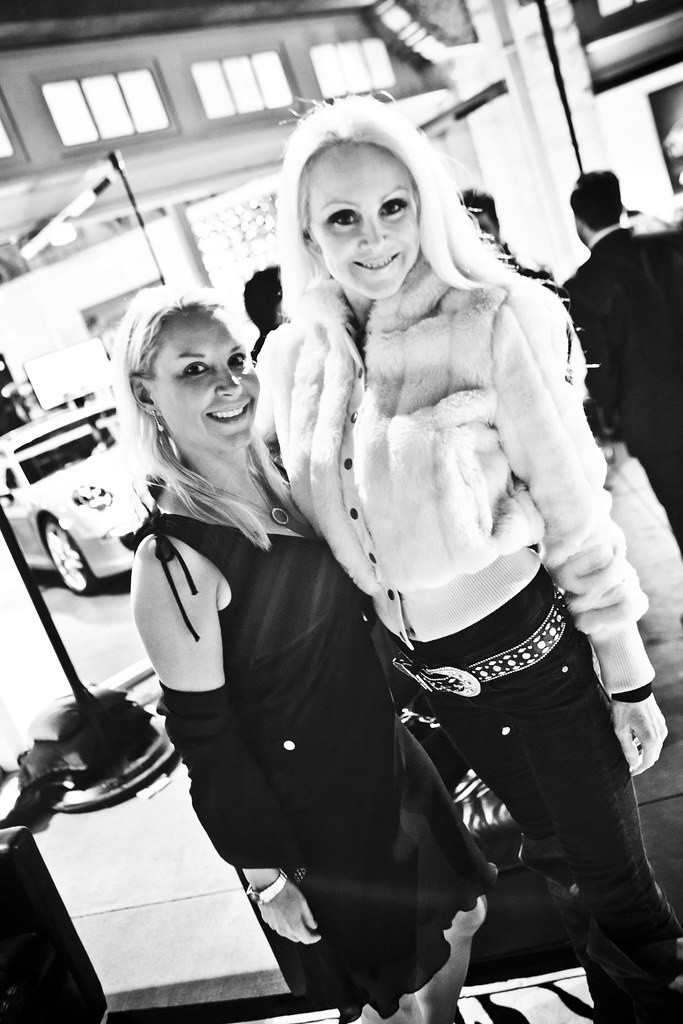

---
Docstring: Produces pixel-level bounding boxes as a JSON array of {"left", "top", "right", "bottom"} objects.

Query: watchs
[{"left": 246, "top": 869, "right": 287, "bottom": 904}]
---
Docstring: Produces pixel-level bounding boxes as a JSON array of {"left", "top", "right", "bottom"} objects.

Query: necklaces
[{"left": 213, "top": 474, "right": 289, "bottom": 525}]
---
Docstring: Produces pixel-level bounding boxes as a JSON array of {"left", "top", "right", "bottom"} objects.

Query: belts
[{"left": 393, "top": 584, "right": 569, "bottom": 698}]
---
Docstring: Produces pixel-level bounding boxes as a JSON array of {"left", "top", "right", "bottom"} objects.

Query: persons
[
  {"left": 459, "top": 188, "right": 556, "bottom": 292},
  {"left": 558, "top": 171, "right": 683, "bottom": 548},
  {"left": 112, "top": 285, "right": 499, "bottom": 1024},
  {"left": 244, "top": 267, "right": 289, "bottom": 361},
  {"left": 247, "top": 102, "right": 683, "bottom": 1024}
]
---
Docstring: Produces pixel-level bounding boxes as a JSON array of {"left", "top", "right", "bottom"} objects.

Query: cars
[{"left": 2, "top": 400, "right": 158, "bottom": 594}]
